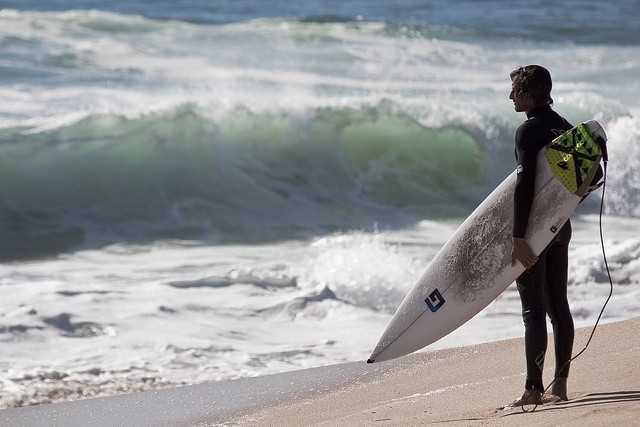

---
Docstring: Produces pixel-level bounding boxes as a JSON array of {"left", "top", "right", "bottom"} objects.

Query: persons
[{"left": 500, "top": 63, "right": 604, "bottom": 409}]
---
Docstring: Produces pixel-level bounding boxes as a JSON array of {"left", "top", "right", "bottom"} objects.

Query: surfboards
[{"left": 367, "top": 119, "right": 608, "bottom": 364}]
